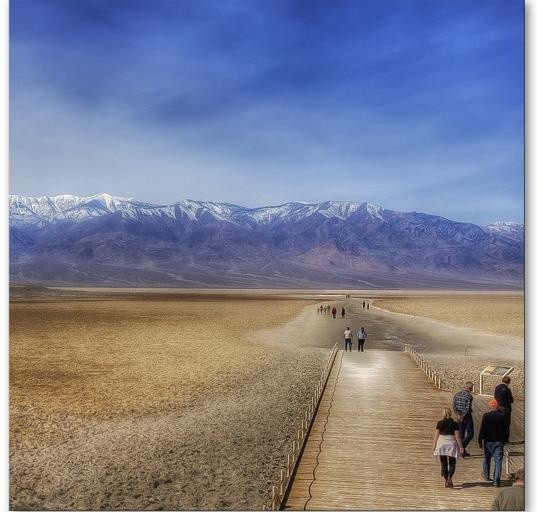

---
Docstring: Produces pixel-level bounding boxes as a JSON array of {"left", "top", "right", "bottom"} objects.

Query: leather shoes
[
  {"left": 491, "top": 482, "right": 499, "bottom": 487},
  {"left": 480, "top": 472, "right": 489, "bottom": 481}
]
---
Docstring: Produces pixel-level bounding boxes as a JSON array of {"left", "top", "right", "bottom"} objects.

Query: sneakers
[
  {"left": 461, "top": 450, "right": 470, "bottom": 458},
  {"left": 445, "top": 479, "right": 453, "bottom": 488}
]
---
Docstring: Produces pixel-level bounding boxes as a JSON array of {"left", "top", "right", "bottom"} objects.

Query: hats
[{"left": 488, "top": 399, "right": 498, "bottom": 407}]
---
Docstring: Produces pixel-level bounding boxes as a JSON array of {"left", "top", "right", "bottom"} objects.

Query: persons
[
  {"left": 320, "top": 305, "right": 322, "bottom": 314},
  {"left": 431, "top": 407, "right": 465, "bottom": 488},
  {"left": 331, "top": 306, "right": 336, "bottom": 318},
  {"left": 492, "top": 376, "right": 513, "bottom": 443},
  {"left": 362, "top": 300, "right": 365, "bottom": 310},
  {"left": 340, "top": 306, "right": 345, "bottom": 319},
  {"left": 477, "top": 399, "right": 509, "bottom": 487},
  {"left": 326, "top": 304, "right": 330, "bottom": 314},
  {"left": 317, "top": 307, "right": 319, "bottom": 312},
  {"left": 451, "top": 381, "right": 474, "bottom": 458},
  {"left": 366, "top": 303, "right": 368, "bottom": 310},
  {"left": 343, "top": 327, "right": 353, "bottom": 351},
  {"left": 323, "top": 307, "right": 326, "bottom": 313},
  {"left": 486, "top": 467, "right": 524, "bottom": 511},
  {"left": 356, "top": 326, "right": 367, "bottom": 353}
]
